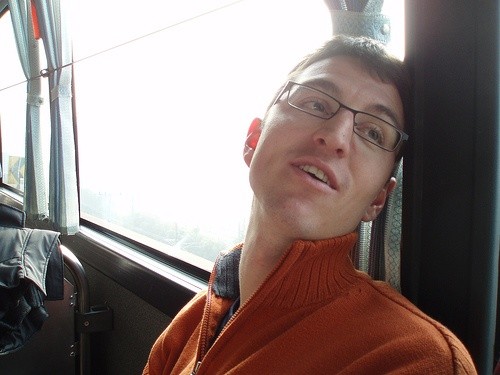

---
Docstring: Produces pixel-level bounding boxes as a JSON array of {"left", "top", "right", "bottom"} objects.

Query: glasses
[{"left": 266, "top": 81, "right": 409, "bottom": 160}]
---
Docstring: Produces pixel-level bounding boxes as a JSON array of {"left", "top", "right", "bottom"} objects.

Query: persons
[{"left": 143, "top": 34, "right": 477, "bottom": 375}]
